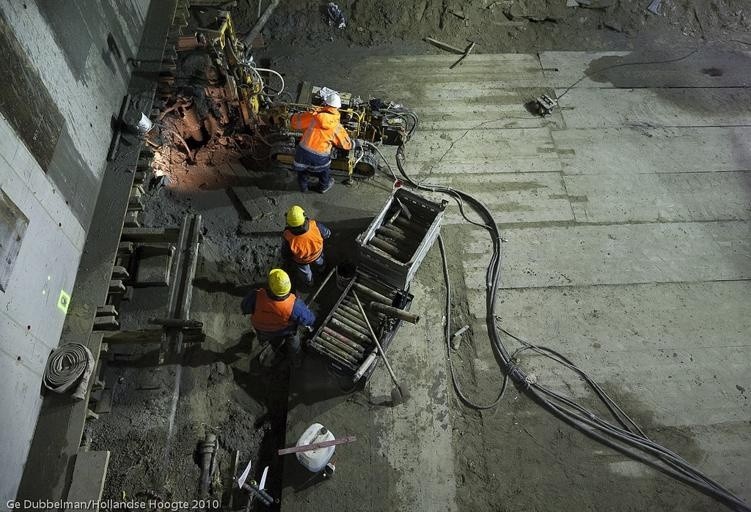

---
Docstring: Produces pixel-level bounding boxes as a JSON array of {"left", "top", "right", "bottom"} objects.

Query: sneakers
[{"left": 302, "top": 177, "right": 335, "bottom": 194}]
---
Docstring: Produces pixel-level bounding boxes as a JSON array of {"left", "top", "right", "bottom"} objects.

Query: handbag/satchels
[{"left": 255, "top": 338, "right": 284, "bottom": 368}]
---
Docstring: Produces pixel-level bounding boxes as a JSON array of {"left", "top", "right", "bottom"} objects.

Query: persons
[
  {"left": 240, "top": 268, "right": 316, "bottom": 359},
  {"left": 290, "top": 94, "right": 364, "bottom": 193},
  {"left": 281, "top": 206, "right": 331, "bottom": 288}
]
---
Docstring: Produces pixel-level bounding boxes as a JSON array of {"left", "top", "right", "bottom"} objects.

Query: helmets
[
  {"left": 326, "top": 94, "right": 342, "bottom": 108},
  {"left": 286, "top": 206, "right": 306, "bottom": 228},
  {"left": 268, "top": 268, "right": 293, "bottom": 298}
]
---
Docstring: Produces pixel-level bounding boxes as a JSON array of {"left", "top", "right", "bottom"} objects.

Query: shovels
[{"left": 352, "top": 289, "right": 411, "bottom": 407}]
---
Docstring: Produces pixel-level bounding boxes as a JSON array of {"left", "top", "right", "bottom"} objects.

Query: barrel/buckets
[
  {"left": 295, "top": 423, "right": 336, "bottom": 474},
  {"left": 125, "top": 110, "right": 155, "bottom": 134}
]
